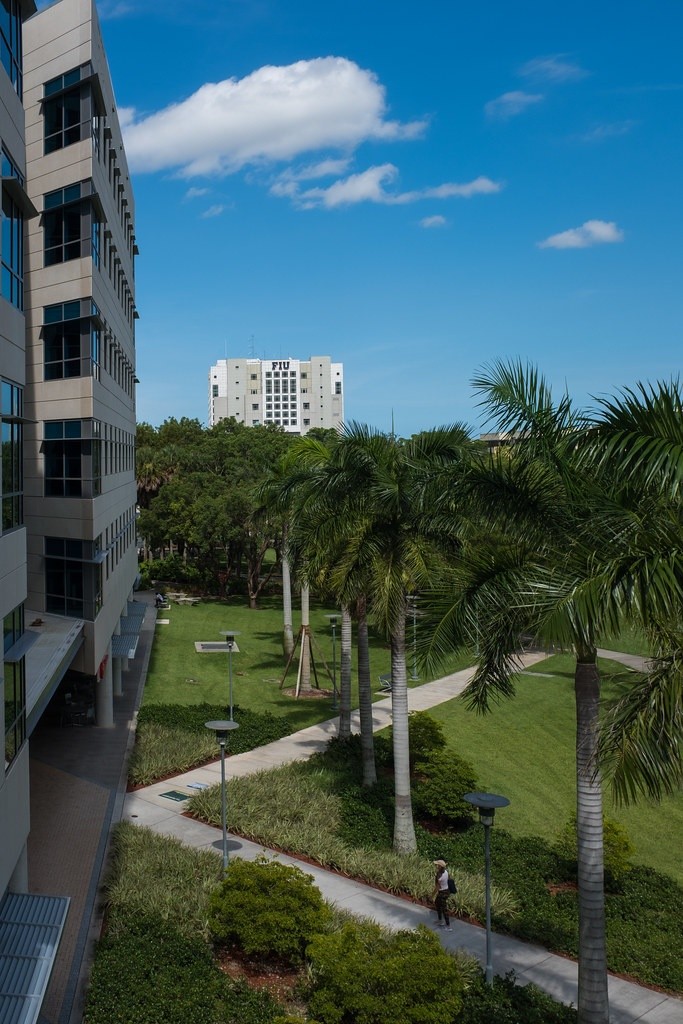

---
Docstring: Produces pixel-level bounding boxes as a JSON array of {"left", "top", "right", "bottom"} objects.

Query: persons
[
  {"left": 155, "top": 592, "right": 163, "bottom": 607},
  {"left": 429, "top": 860, "right": 453, "bottom": 931}
]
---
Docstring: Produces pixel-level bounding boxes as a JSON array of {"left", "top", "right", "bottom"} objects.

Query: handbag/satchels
[{"left": 447, "top": 877, "right": 457, "bottom": 894}]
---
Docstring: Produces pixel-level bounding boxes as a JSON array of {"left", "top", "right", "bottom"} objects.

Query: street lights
[
  {"left": 462, "top": 791, "right": 512, "bottom": 992},
  {"left": 205, "top": 721, "right": 239, "bottom": 881},
  {"left": 220, "top": 630, "right": 241, "bottom": 722},
  {"left": 406, "top": 595, "right": 423, "bottom": 681},
  {"left": 324, "top": 614, "right": 341, "bottom": 711}
]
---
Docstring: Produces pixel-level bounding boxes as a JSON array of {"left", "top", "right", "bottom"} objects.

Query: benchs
[
  {"left": 379, "top": 673, "right": 392, "bottom": 692},
  {"left": 160, "top": 602, "right": 167, "bottom": 608}
]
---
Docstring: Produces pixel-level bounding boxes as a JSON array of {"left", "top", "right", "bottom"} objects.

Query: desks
[
  {"left": 165, "top": 592, "right": 199, "bottom": 605},
  {"left": 62, "top": 694, "right": 91, "bottom": 727}
]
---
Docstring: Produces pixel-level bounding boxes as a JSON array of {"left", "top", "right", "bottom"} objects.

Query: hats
[{"left": 433, "top": 860, "right": 447, "bottom": 868}]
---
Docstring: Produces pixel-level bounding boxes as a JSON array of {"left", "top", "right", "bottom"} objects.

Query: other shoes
[
  {"left": 443, "top": 927, "right": 453, "bottom": 932},
  {"left": 434, "top": 921, "right": 445, "bottom": 926}
]
